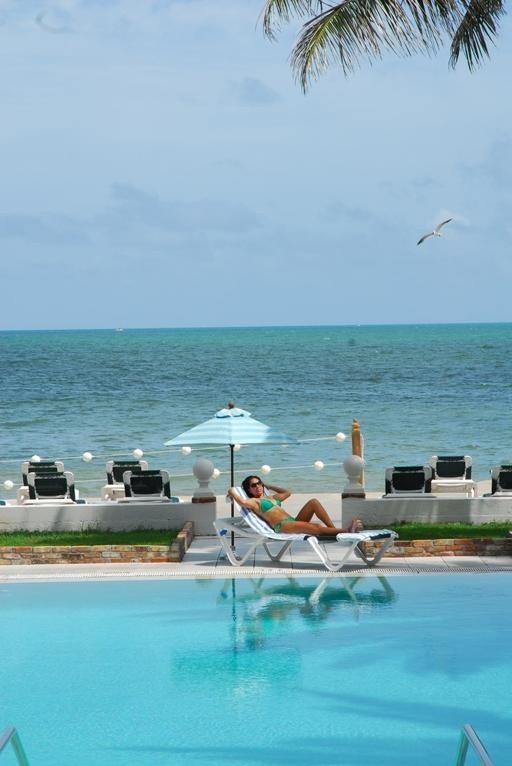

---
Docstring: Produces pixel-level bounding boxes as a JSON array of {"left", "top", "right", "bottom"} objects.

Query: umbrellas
[
  {"left": 352, "top": 416, "right": 364, "bottom": 485},
  {"left": 162, "top": 400, "right": 302, "bottom": 560}
]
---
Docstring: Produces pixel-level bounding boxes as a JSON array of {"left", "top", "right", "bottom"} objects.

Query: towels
[{"left": 226, "top": 486, "right": 311, "bottom": 541}]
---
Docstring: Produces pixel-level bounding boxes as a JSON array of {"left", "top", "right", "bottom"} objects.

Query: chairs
[
  {"left": 16, "top": 461, "right": 179, "bottom": 505},
  {"left": 212, "top": 485, "right": 399, "bottom": 572},
  {"left": 216, "top": 578, "right": 395, "bottom": 621},
  {"left": 384, "top": 455, "right": 512, "bottom": 498}
]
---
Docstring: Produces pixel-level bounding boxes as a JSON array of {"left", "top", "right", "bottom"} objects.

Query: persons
[
  {"left": 228, "top": 476, "right": 364, "bottom": 536},
  {"left": 242, "top": 599, "right": 333, "bottom": 653}
]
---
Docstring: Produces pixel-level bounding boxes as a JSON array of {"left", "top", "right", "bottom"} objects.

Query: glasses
[{"left": 252, "top": 481, "right": 259, "bottom": 487}]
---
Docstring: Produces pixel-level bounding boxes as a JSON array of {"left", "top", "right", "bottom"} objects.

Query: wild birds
[{"left": 417, "top": 217, "right": 453, "bottom": 245}]
